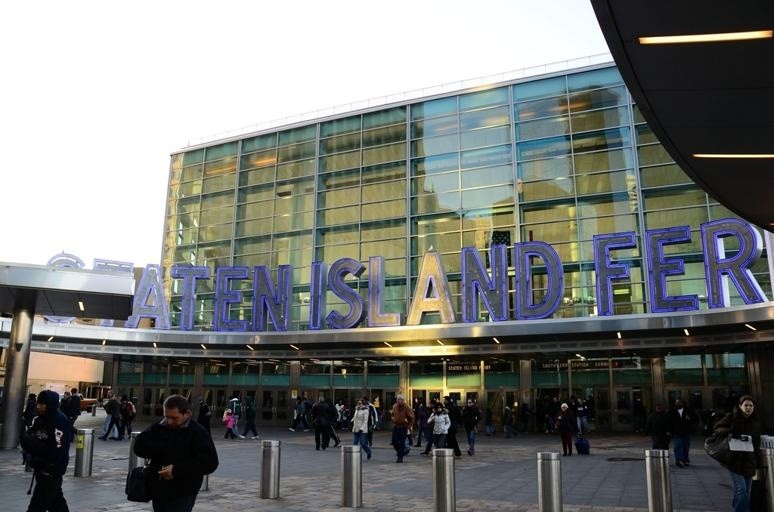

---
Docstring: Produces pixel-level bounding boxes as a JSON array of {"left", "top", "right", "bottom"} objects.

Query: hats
[{"left": 561, "top": 403, "right": 568, "bottom": 408}]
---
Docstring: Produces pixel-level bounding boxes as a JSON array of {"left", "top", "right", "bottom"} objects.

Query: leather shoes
[{"left": 367, "top": 452, "right": 371, "bottom": 458}]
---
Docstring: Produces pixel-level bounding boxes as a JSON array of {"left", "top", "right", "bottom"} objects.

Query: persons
[
  {"left": 23, "top": 391, "right": 72, "bottom": 510},
  {"left": 649, "top": 398, "right": 696, "bottom": 469},
  {"left": 536, "top": 394, "right": 606, "bottom": 456},
  {"left": 198, "top": 396, "right": 259, "bottom": 440},
  {"left": 387, "top": 395, "right": 494, "bottom": 463},
  {"left": 288, "top": 396, "right": 385, "bottom": 459},
  {"left": 712, "top": 394, "right": 763, "bottom": 512},
  {"left": 133, "top": 392, "right": 217, "bottom": 512},
  {"left": 98, "top": 394, "right": 137, "bottom": 442},
  {"left": 22, "top": 394, "right": 40, "bottom": 471},
  {"left": 61, "top": 389, "right": 83, "bottom": 442},
  {"left": 502, "top": 403, "right": 532, "bottom": 439},
  {"left": 632, "top": 396, "right": 647, "bottom": 432}
]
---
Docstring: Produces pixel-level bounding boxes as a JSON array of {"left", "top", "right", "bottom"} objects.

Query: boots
[{"left": 467, "top": 443, "right": 474, "bottom": 455}]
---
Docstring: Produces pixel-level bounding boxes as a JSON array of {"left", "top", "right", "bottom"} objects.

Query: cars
[{"left": 78, "top": 394, "right": 97, "bottom": 412}]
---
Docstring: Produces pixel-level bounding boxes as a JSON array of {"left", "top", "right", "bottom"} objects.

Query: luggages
[{"left": 574, "top": 432, "right": 590, "bottom": 455}]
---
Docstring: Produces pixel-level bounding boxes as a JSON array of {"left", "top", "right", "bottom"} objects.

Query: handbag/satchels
[
  {"left": 125, "top": 464, "right": 152, "bottom": 502},
  {"left": 555, "top": 420, "right": 560, "bottom": 429},
  {"left": 704, "top": 413, "right": 735, "bottom": 464}
]
